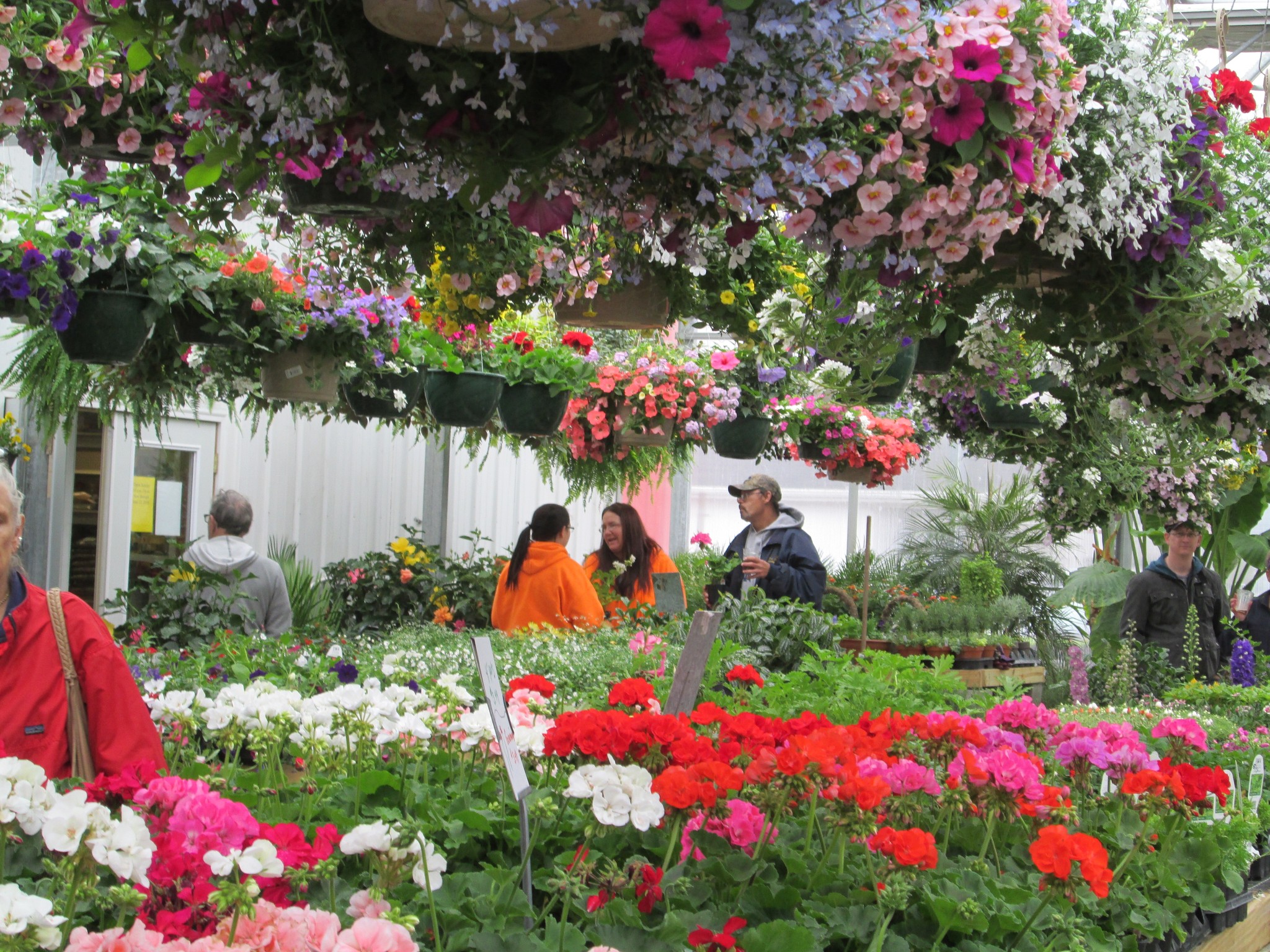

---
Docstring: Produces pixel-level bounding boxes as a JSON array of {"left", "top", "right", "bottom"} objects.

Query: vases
[
  {"left": 823, "top": 453, "right": 871, "bottom": 485},
  {"left": 913, "top": 334, "right": 962, "bottom": 371},
  {"left": 973, "top": 385, "right": 1039, "bottom": 434},
  {"left": 421, "top": 369, "right": 507, "bottom": 430},
  {"left": 363, "top": 0, "right": 627, "bottom": 53},
  {"left": 168, "top": 297, "right": 235, "bottom": 341},
  {"left": 838, "top": 335, "right": 914, "bottom": 400},
  {"left": 53, "top": 288, "right": 154, "bottom": 370},
  {"left": 283, "top": 166, "right": 407, "bottom": 213},
  {"left": 601, "top": 394, "right": 678, "bottom": 443},
  {"left": 256, "top": 336, "right": 340, "bottom": 402},
  {"left": 497, "top": 384, "right": 573, "bottom": 441},
  {"left": 709, "top": 412, "right": 772, "bottom": 460},
  {"left": 339, "top": 365, "right": 424, "bottom": 417},
  {"left": 797, "top": 425, "right": 826, "bottom": 459},
  {"left": 553, "top": 281, "right": 670, "bottom": 329},
  {"left": 64, "top": 111, "right": 172, "bottom": 166}
]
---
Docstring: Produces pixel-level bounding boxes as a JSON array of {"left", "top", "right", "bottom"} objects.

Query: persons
[
  {"left": 1119, "top": 521, "right": 1230, "bottom": 684},
  {"left": 582, "top": 503, "right": 688, "bottom": 621},
  {"left": 703, "top": 475, "right": 826, "bottom": 612},
  {"left": 1230, "top": 551, "right": 1270, "bottom": 657},
  {"left": 0, "top": 449, "right": 169, "bottom": 779},
  {"left": 492, "top": 504, "right": 605, "bottom": 630},
  {"left": 174, "top": 488, "right": 292, "bottom": 640}
]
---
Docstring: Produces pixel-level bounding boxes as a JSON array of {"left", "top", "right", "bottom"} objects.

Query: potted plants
[{"left": 838, "top": 612, "right": 1013, "bottom": 669}]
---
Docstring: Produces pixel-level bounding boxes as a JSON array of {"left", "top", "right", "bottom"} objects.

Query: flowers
[
  {"left": 0, "top": 0, "right": 1270, "bottom": 545},
  {"left": 2, "top": 627, "right": 1270, "bottom": 951}
]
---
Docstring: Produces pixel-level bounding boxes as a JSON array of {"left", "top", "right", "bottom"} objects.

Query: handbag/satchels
[{"left": 47, "top": 587, "right": 125, "bottom": 814}]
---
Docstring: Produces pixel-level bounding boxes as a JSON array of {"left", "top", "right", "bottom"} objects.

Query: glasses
[
  {"left": 601, "top": 523, "right": 622, "bottom": 532},
  {"left": 204, "top": 514, "right": 220, "bottom": 528},
  {"left": 566, "top": 526, "right": 574, "bottom": 533},
  {"left": 1169, "top": 531, "right": 1200, "bottom": 539},
  {"left": 741, "top": 492, "right": 763, "bottom": 502}
]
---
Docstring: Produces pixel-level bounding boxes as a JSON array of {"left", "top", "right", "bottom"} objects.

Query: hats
[
  {"left": 728, "top": 474, "right": 782, "bottom": 501},
  {"left": 1164, "top": 516, "right": 1203, "bottom": 533}
]
